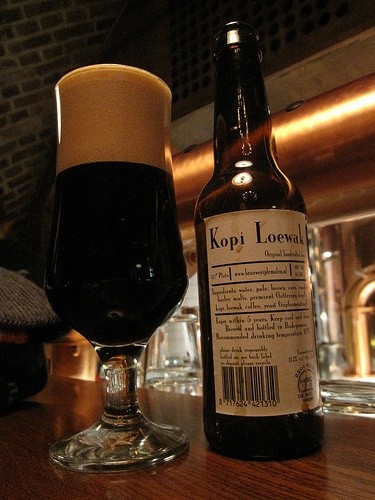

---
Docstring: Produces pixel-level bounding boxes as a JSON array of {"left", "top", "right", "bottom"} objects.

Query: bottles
[{"left": 193, "top": 20, "right": 325, "bottom": 461}]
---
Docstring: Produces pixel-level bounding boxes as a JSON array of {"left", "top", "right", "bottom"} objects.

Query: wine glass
[{"left": 43, "top": 63, "right": 190, "bottom": 473}]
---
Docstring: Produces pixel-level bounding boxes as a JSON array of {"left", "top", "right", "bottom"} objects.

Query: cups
[{"left": 145, "top": 315, "right": 201, "bottom": 397}]
